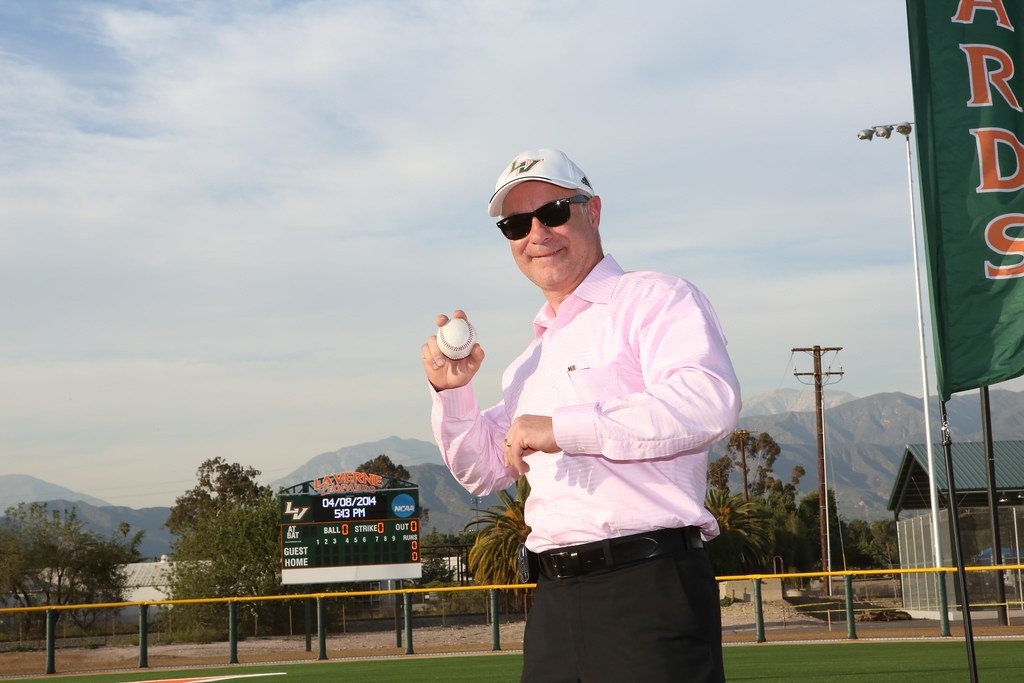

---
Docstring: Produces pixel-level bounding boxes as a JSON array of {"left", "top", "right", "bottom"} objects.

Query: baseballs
[{"left": 435, "top": 317, "right": 476, "bottom": 360}]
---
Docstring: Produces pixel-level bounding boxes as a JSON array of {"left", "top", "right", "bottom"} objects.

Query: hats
[{"left": 487, "top": 149, "right": 594, "bottom": 217}]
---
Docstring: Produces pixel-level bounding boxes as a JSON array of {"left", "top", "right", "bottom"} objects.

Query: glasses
[{"left": 496, "top": 194, "right": 591, "bottom": 241}]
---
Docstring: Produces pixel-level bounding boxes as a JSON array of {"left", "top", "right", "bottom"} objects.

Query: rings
[{"left": 505, "top": 437, "right": 511, "bottom": 447}]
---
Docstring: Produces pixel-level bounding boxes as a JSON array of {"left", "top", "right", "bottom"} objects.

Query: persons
[{"left": 419, "top": 149, "right": 741, "bottom": 683}]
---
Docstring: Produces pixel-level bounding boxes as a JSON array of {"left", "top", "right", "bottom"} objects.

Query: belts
[{"left": 536, "top": 528, "right": 704, "bottom": 583}]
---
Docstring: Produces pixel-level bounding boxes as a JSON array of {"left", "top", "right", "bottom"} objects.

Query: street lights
[{"left": 857, "top": 120, "right": 954, "bottom": 640}]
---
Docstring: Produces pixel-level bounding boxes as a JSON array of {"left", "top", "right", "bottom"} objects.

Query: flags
[{"left": 910, "top": 1, "right": 1023, "bottom": 400}]
